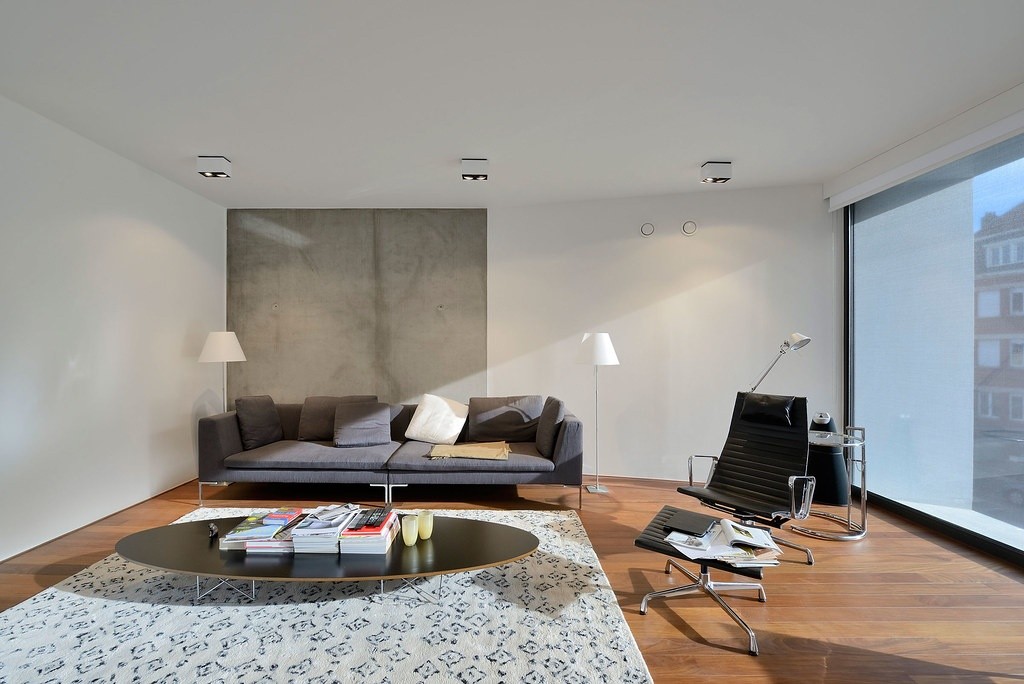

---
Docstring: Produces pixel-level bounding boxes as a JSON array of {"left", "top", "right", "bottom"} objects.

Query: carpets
[{"left": 0, "top": 507, "right": 654, "bottom": 684}]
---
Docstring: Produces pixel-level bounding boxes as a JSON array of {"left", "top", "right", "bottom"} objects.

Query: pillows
[
  {"left": 298, "top": 395, "right": 378, "bottom": 439},
  {"left": 405, "top": 393, "right": 469, "bottom": 446},
  {"left": 469, "top": 396, "right": 542, "bottom": 442},
  {"left": 334, "top": 403, "right": 391, "bottom": 447},
  {"left": 235, "top": 395, "right": 284, "bottom": 450},
  {"left": 535, "top": 396, "right": 565, "bottom": 459}
]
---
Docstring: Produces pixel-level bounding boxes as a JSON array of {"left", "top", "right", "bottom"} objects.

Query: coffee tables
[{"left": 115, "top": 513, "right": 540, "bottom": 603}]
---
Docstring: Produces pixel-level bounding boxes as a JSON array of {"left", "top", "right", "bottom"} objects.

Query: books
[
  {"left": 662, "top": 509, "right": 784, "bottom": 567},
  {"left": 220, "top": 507, "right": 311, "bottom": 553},
  {"left": 292, "top": 506, "right": 359, "bottom": 554},
  {"left": 339, "top": 508, "right": 401, "bottom": 554}
]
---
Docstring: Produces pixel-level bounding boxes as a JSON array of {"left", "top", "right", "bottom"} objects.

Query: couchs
[{"left": 198, "top": 403, "right": 583, "bottom": 510}]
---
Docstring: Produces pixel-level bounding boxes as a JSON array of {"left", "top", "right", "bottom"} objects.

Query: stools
[{"left": 635, "top": 504, "right": 772, "bottom": 655}]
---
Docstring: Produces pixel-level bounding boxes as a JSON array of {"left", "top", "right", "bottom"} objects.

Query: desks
[{"left": 791, "top": 427, "right": 867, "bottom": 541}]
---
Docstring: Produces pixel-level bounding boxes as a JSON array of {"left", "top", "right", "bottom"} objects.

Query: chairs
[{"left": 676, "top": 391, "right": 816, "bottom": 565}]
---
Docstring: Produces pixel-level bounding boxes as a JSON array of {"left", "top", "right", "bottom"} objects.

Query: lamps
[
  {"left": 461, "top": 159, "right": 488, "bottom": 181},
  {"left": 701, "top": 162, "right": 732, "bottom": 184},
  {"left": 577, "top": 332, "right": 620, "bottom": 493},
  {"left": 198, "top": 332, "right": 247, "bottom": 486},
  {"left": 197, "top": 156, "right": 232, "bottom": 179},
  {"left": 750, "top": 332, "right": 812, "bottom": 393}
]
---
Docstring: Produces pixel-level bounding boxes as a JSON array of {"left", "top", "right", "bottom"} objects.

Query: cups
[
  {"left": 402, "top": 515, "right": 418, "bottom": 547},
  {"left": 418, "top": 510, "right": 433, "bottom": 540}
]
